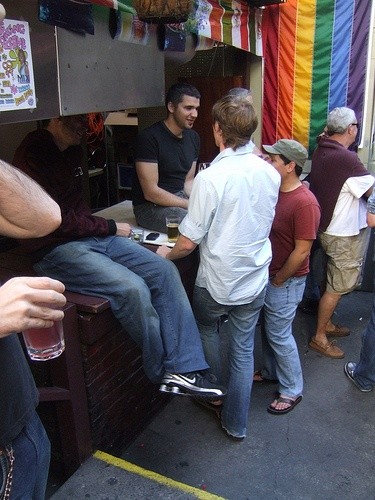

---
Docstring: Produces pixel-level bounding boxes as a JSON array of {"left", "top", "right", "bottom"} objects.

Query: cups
[
  {"left": 198, "top": 162, "right": 210, "bottom": 172},
  {"left": 131, "top": 229, "right": 144, "bottom": 246},
  {"left": 21, "top": 302, "right": 66, "bottom": 362},
  {"left": 166, "top": 215, "right": 180, "bottom": 242}
]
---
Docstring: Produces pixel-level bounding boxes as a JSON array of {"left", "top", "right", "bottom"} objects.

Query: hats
[{"left": 262, "top": 139, "right": 308, "bottom": 167}]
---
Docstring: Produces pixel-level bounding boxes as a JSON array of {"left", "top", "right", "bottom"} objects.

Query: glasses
[{"left": 352, "top": 123, "right": 359, "bottom": 128}]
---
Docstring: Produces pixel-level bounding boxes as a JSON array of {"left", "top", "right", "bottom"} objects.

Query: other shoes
[
  {"left": 192, "top": 398, "right": 223, "bottom": 411},
  {"left": 215, "top": 411, "right": 244, "bottom": 441}
]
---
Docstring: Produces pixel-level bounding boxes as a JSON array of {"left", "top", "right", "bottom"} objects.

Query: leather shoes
[
  {"left": 309, "top": 336, "right": 344, "bottom": 359},
  {"left": 325, "top": 326, "right": 350, "bottom": 337}
]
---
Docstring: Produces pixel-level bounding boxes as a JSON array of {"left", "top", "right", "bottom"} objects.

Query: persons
[
  {"left": 155, "top": 94, "right": 282, "bottom": 441},
  {"left": 252, "top": 138, "right": 320, "bottom": 416},
  {"left": 0, "top": 159, "right": 66, "bottom": 500},
  {"left": 18, "top": 48, "right": 29, "bottom": 84},
  {"left": 10, "top": 112, "right": 227, "bottom": 412},
  {"left": 344, "top": 187, "right": 375, "bottom": 392},
  {"left": 308, "top": 106, "right": 375, "bottom": 359},
  {"left": 131, "top": 83, "right": 202, "bottom": 287}
]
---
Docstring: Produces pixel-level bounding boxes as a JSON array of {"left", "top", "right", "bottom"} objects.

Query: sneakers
[
  {"left": 345, "top": 361, "right": 371, "bottom": 391},
  {"left": 202, "top": 372, "right": 218, "bottom": 384},
  {"left": 158, "top": 372, "right": 227, "bottom": 397}
]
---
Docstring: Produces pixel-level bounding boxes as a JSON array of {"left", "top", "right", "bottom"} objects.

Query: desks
[{"left": 0, "top": 198, "right": 212, "bottom": 482}]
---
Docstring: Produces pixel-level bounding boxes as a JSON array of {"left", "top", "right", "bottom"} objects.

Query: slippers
[
  {"left": 267, "top": 396, "right": 302, "bottom": 414},
  {"left": 252, "top": 370, "right": 278, "bottom": 384}
]
